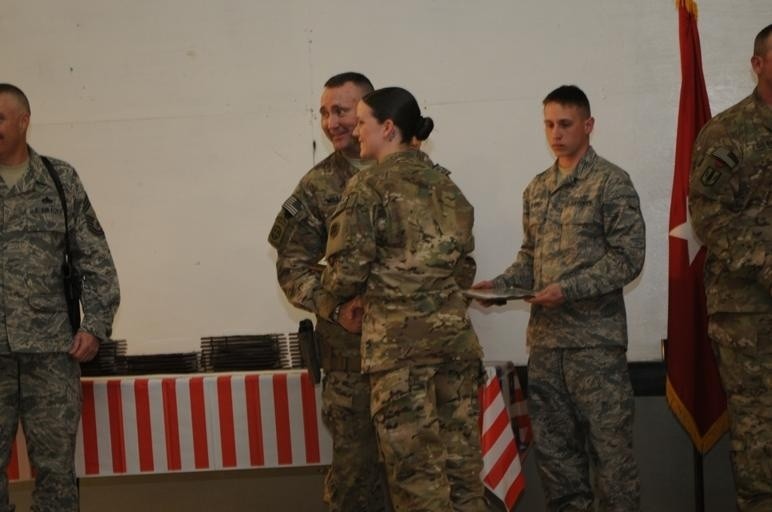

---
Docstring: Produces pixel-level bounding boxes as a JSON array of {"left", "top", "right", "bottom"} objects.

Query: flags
[{"left": 663, "top": 0, "right": 732, "bottom": 456}]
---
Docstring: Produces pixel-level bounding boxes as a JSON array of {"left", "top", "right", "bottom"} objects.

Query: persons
[
  {"left": 318, "top": 85, "right": 489, "bottom": 512},
  {"left": 687, "top": 23, "right": 772, "bottom": 512},
  {"left": 265, "top": 72, "right": 378, "bottom": 512},
  {"left": 0, "top": 82, "right": 122, "bottom": 512},
  {"left": 471, "top": 82, "right": 646, "bottom": 512}
]
